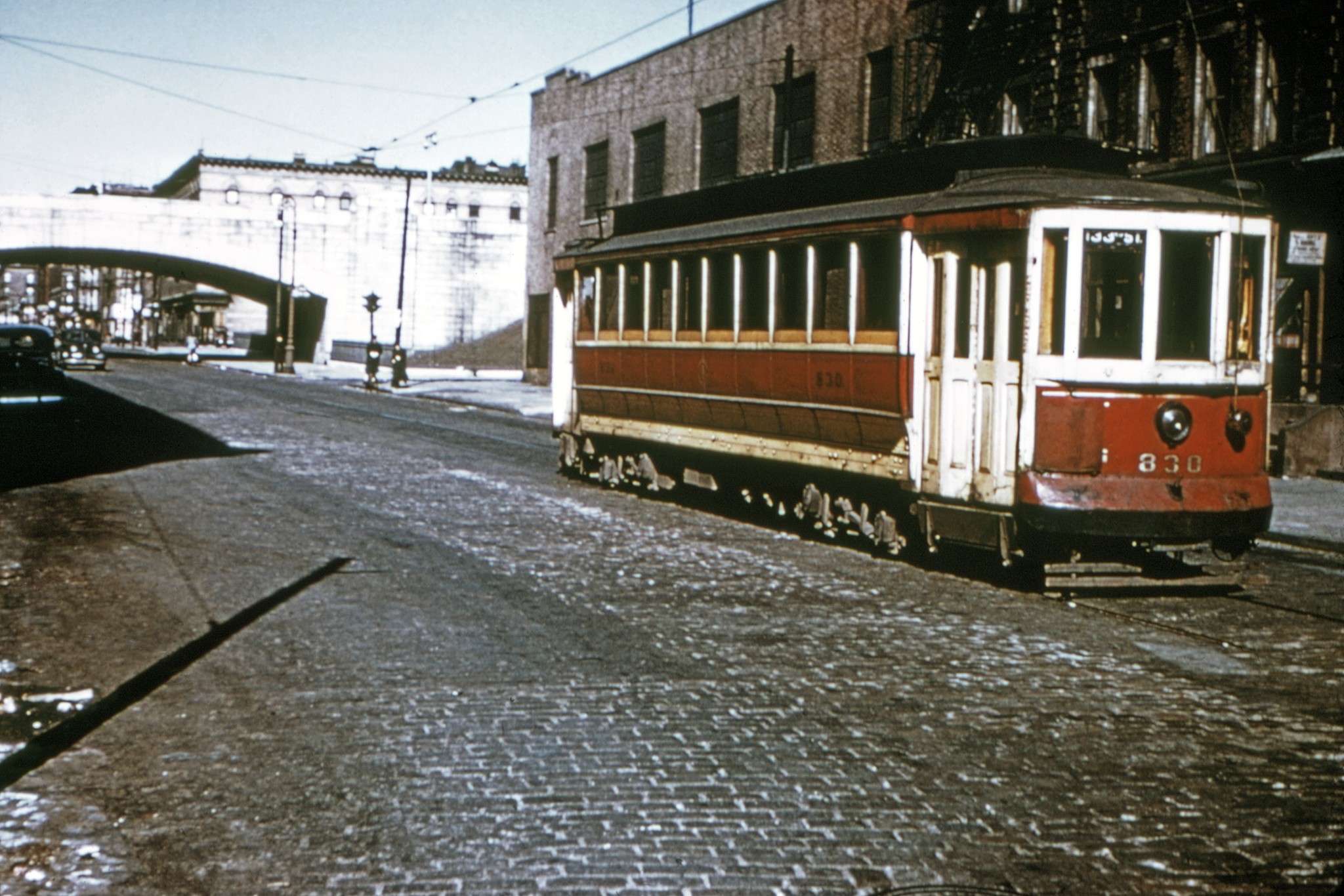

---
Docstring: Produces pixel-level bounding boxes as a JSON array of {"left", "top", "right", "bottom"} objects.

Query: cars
[
  {"left": 57, "top": 327, "right": 107, "bottom": 371},
  {"left": 0, "top": 322, "right": 66, "bottom": 397}
]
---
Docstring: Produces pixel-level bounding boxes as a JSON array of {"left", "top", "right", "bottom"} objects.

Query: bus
[{"left": 550, "top": 124, "right": 1276, "bottom": 595}]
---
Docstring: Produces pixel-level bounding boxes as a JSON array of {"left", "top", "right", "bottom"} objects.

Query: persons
[
  {"left": 186, "top": 333, "right": 198, "bottom": 354},
  {"left": 390, "top": 343, "right": 408, "bottom": 387},
  {"left": 366, "top": 335, "right": 383, "bottom": 384}
]
